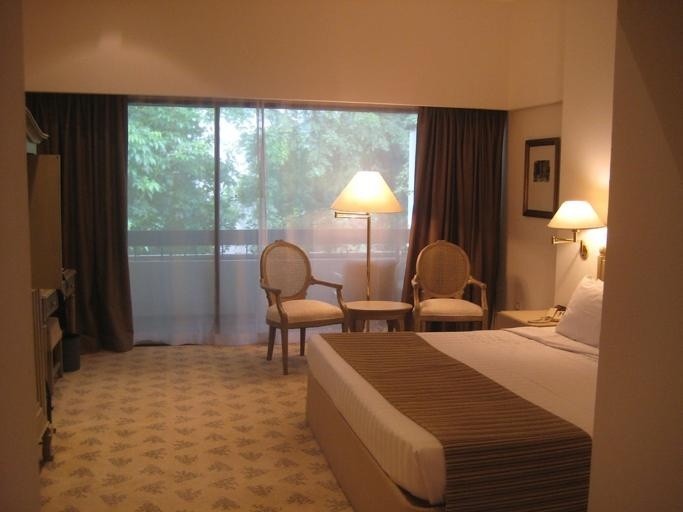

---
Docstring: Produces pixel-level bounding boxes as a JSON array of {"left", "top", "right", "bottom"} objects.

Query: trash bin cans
[{"left": 62, "top": 333, "right": 80, "bottom": 372}]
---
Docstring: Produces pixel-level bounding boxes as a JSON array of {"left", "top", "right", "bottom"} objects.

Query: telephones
[{"left": 545, "top": 305, "right": 568, "bottom": 322}]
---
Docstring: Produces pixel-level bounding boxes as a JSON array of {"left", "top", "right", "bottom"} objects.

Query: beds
[{"left": 305, "top": 244, "right": 607, "bottom": 512}]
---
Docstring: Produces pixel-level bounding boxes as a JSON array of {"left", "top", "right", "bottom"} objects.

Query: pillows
[{"left": 554, "top": 273, "right": 604, "bottom": 349}]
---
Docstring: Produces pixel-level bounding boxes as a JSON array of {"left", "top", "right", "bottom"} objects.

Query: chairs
[
  {"left": 258, "top": 238, "right": 347, "bottom": 375},
  {"left": 410, "top": 239, "right": 489, "bottom": 332}
]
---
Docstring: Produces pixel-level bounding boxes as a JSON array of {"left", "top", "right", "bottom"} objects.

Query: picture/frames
[{"left": 521, "top": 136, "right": 560, "bottom": 219}]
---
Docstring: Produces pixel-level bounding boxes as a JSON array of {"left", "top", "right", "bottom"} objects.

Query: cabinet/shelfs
[{"left": 22, "top": 107, "right": 77, "bottom": 459}]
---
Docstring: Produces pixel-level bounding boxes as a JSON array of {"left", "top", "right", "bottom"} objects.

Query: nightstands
[{"left": 493, "top": 308, "right": 563, "bottom": 331}]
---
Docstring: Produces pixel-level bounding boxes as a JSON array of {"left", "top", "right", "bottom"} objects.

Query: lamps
[
  {"left": 329, "top": 171, "right": 402, "bottom": 299},
  {"left": 545, "top": 200, "right": 605, "bottom": 245}
]
